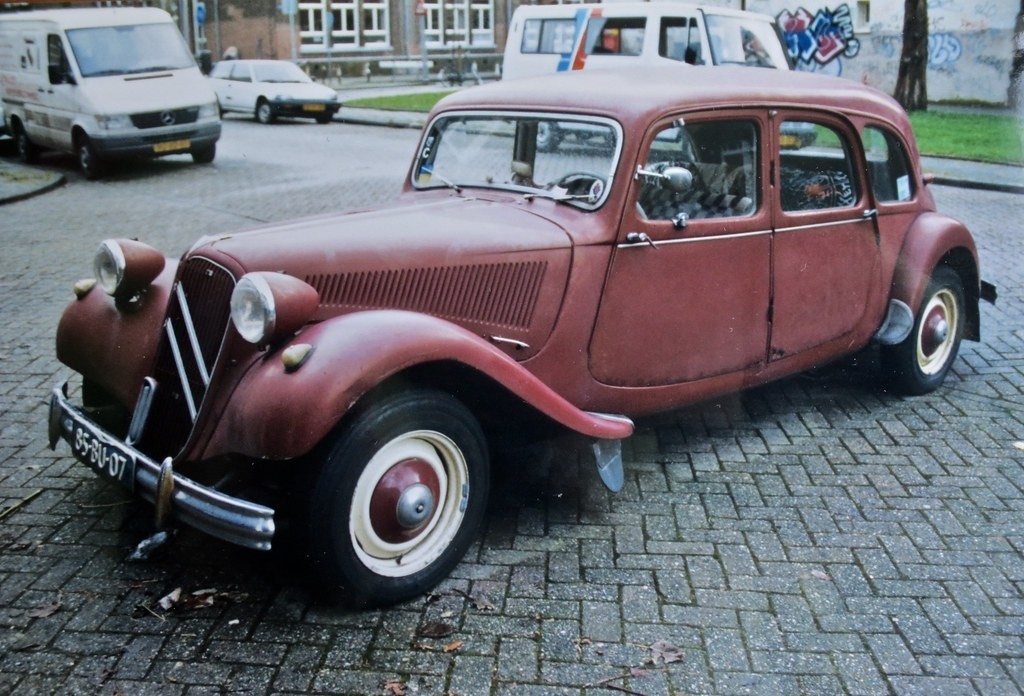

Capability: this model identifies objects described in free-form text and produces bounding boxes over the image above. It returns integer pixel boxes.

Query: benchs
[648,160,854,211]
[566,177,752,220]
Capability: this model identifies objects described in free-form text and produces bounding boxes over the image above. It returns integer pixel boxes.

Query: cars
[208,58,343,124]
[45,65,997,602]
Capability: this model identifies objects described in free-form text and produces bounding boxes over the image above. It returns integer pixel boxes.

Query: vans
[503,4,791,79]
[1,7,222,179]
